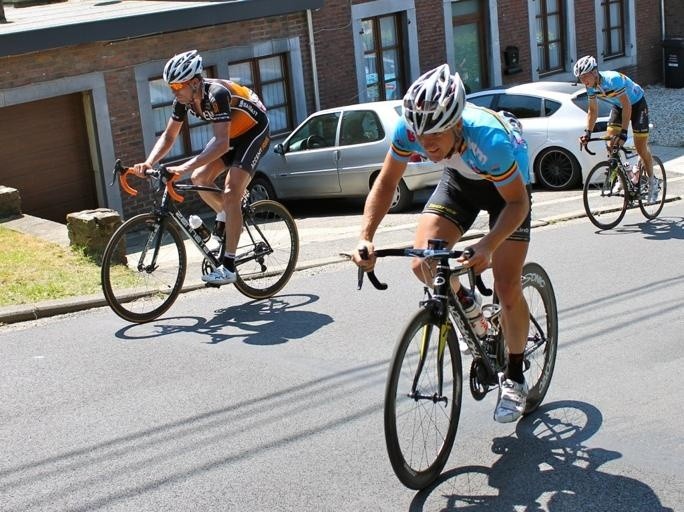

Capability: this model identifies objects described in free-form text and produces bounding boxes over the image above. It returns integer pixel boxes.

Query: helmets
[574,55,598,77]
[162,48,202,85]
[402,63,466,136]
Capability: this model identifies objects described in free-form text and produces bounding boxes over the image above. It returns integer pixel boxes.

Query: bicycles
[100,158,298,323]
[338,238,558,490]
[580,135,667,230]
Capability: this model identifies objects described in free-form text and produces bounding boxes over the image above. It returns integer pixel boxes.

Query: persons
[570,54,660,200]
[352,61,534,426]
[127,48,270,287]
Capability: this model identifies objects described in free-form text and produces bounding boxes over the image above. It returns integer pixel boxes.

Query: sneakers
[495,374,529,423]
[611,182,624,194]
[648,178,659,205]
[202,265,237,284]
[459,290,483,355]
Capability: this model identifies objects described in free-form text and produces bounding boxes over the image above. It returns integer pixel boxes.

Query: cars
[242,97,527,220]
[463,81,653,192]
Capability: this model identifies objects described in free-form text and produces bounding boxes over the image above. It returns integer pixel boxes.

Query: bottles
[622,162,634,180]
[632,163,639,183]
[214,212,227,240]
[462,293,490,341]
[187,214,212,241]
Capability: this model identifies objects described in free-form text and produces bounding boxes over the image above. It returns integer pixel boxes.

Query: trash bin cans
[664,37,684,88]
